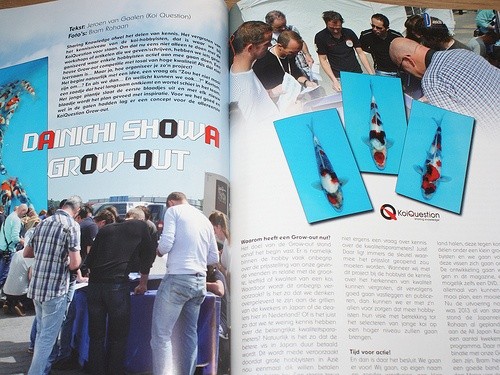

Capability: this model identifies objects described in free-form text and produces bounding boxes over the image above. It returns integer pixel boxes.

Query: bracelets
[304,80,307,88]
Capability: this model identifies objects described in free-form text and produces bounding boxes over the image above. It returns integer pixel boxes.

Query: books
[0,0,500,375]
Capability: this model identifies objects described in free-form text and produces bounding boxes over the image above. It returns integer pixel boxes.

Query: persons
[230,10,319,117]
[466,10,500,68]
[22,194,82,375]
[206,212,231,335]
[131,205,158,272]
[359,13,404,78]
[84,208,158,375]
[150,192,219,375]
[403,15,474,99]
[0,204,118,353]
[313,11,373,94]
[49,209,115,370]
[389,38,500,134]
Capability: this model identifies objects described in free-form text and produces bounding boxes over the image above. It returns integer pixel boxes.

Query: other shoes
[15,303,27,316]
[3,302,10,314]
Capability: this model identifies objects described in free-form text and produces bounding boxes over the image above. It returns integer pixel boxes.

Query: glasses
[397,54,410,73]
[371,24,385,30]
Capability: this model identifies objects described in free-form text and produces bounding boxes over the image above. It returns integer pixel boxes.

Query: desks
[62,281,224,375]
